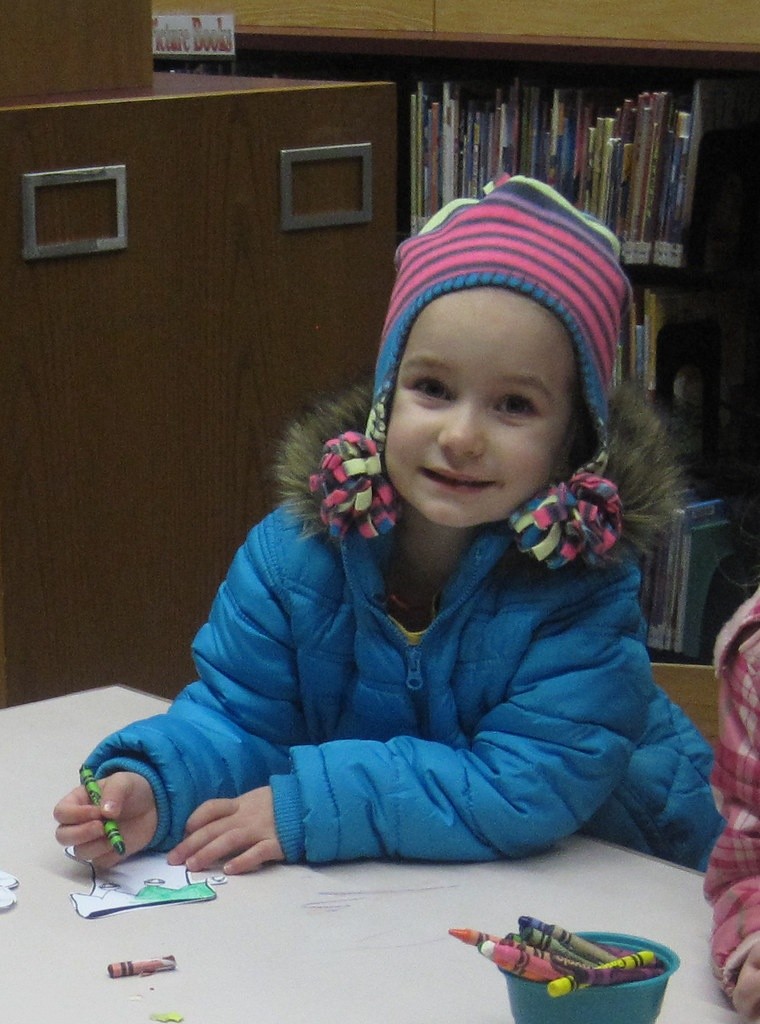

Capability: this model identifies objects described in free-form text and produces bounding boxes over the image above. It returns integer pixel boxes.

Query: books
[409,72,728,654]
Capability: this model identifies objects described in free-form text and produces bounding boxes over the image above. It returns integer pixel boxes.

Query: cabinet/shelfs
[390,41,760,743]
[1,77,390,703]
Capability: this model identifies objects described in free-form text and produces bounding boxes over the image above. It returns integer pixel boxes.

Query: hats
[306,172,624,570]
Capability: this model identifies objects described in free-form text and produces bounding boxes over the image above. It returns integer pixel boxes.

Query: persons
[702,580,760,1024]
[54,172,726,876]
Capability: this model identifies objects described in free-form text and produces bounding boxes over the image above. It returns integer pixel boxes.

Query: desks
[0,679,759,1024]
